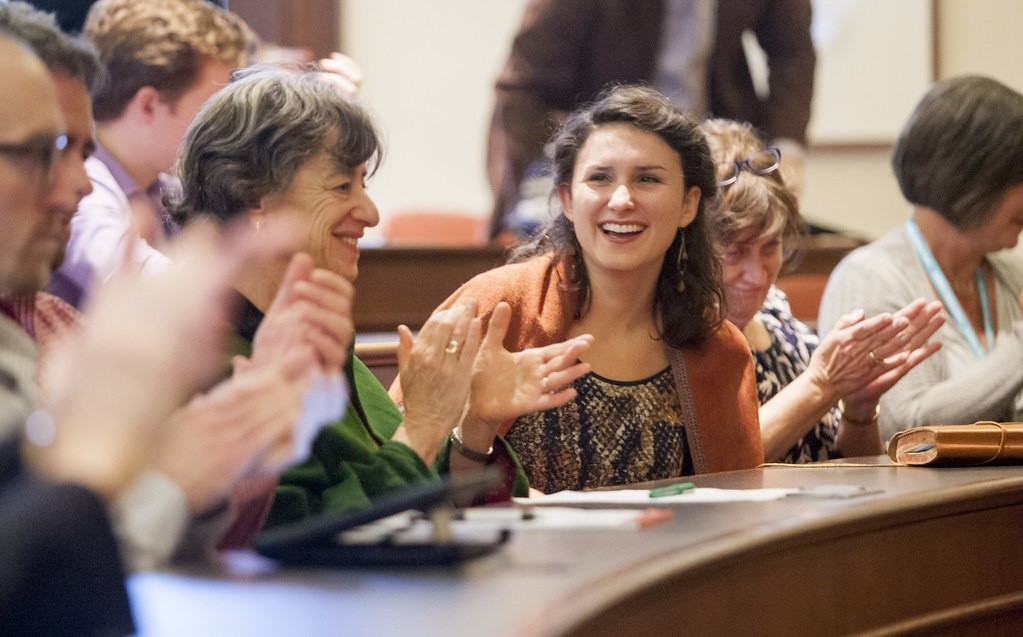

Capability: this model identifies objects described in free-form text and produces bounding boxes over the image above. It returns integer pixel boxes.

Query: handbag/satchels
[888,421,1023,466]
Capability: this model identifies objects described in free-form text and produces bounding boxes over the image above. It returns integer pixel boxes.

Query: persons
[815,73,1023,443]
[493,0,817,247]
[390,85,762,495]
[0,0,355,637]
[162,71,594,563]
[693,116,946,462]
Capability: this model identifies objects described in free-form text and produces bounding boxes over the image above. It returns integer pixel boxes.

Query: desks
[107,241,1023,637]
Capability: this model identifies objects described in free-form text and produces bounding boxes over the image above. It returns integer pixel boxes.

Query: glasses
[0,134,69,190]
[715,146,781,186]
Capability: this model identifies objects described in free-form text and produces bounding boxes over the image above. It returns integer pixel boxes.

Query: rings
[869,351,880,366]
[444,342,458,353]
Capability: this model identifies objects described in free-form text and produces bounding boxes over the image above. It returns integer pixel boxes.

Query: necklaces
[904,220,994,366]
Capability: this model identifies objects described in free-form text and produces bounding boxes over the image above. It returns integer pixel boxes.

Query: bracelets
[450,429,493,463]
[837,399,880,425]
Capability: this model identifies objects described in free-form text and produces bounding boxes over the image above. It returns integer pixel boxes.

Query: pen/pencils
[421,509,536,521]
[648,482,694,498]
